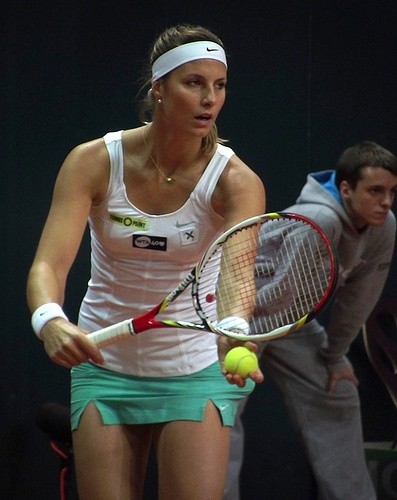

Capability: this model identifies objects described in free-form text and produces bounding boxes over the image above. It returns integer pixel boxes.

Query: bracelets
[215,317,250,344]
[31,303,68,340]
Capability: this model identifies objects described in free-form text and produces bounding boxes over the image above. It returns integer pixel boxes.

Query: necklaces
[143,122,203,182]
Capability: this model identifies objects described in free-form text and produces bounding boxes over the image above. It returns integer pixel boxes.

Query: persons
[223,141,397,500]
[27,25,265,500]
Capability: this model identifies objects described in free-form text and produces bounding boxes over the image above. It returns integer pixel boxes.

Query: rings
[351,376,352,380]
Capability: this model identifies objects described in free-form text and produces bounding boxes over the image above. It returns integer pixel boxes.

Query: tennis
[223,347,258,379]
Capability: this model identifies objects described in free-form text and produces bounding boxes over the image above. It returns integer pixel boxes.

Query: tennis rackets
[84,212,341,352]
